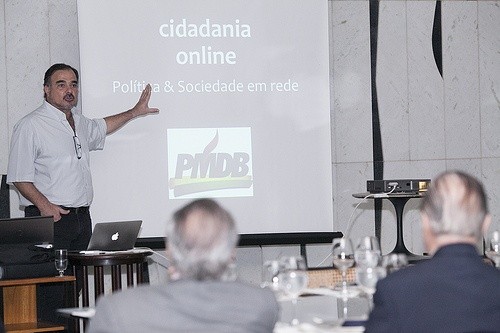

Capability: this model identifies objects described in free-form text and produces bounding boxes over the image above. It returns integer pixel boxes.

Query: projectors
[366,179,431,193]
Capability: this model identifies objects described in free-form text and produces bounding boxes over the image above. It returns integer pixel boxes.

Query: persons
[84,199,280,333]
[364,169,500,333]
[6,64,160,333]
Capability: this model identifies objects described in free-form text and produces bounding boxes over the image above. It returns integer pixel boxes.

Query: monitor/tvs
[0,215,53,265]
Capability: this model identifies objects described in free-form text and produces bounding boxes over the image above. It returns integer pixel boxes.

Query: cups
[489,230,500,252]
[354,235,380,269]
[260,260,281,298]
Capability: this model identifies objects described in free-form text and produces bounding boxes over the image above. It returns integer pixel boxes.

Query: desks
[59,250,155,333]
[0,274,77,333]
[353,193,428,259]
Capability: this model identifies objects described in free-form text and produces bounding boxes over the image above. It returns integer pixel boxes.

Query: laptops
[68,221,142,254]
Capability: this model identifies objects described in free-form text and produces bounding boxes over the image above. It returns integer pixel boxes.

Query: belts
[68,206,89,214]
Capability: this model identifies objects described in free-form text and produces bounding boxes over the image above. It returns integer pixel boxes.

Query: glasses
[73,135,82,160]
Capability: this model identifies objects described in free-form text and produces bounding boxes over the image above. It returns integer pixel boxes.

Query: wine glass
[280,256,312,329]
[332,238,358,296]
[355,250,386,311]
[54,249,68,277]
